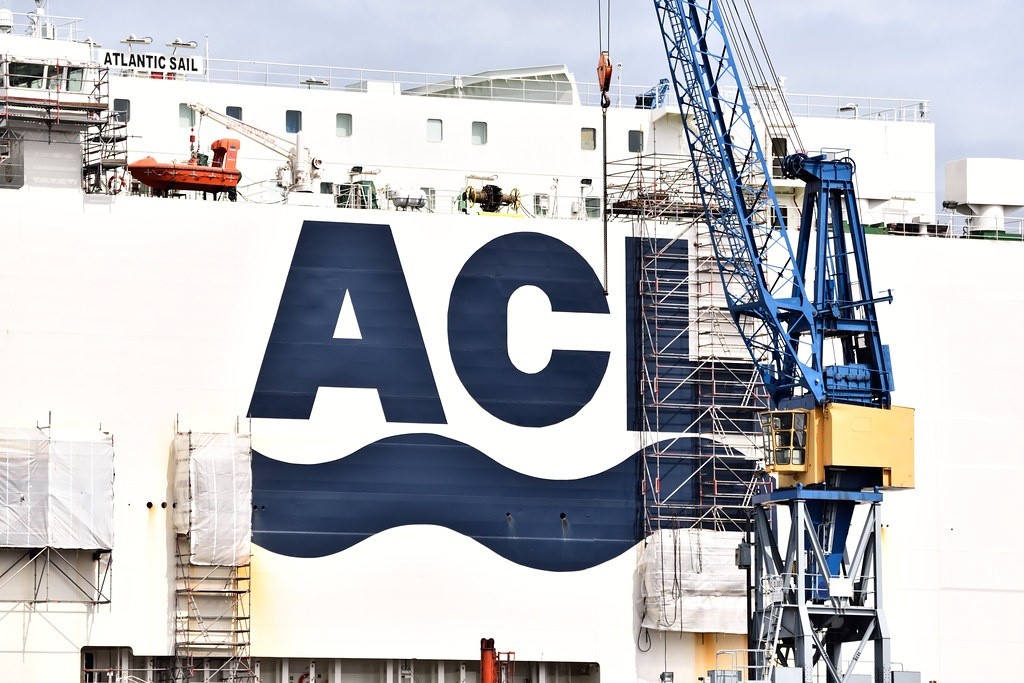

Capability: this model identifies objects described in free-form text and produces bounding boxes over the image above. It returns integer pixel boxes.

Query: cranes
[592,1,924,682]
[190,101,322,193]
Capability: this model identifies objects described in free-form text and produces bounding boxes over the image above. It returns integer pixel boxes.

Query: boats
[128,139,242,192]
[0,0,1024,683]
